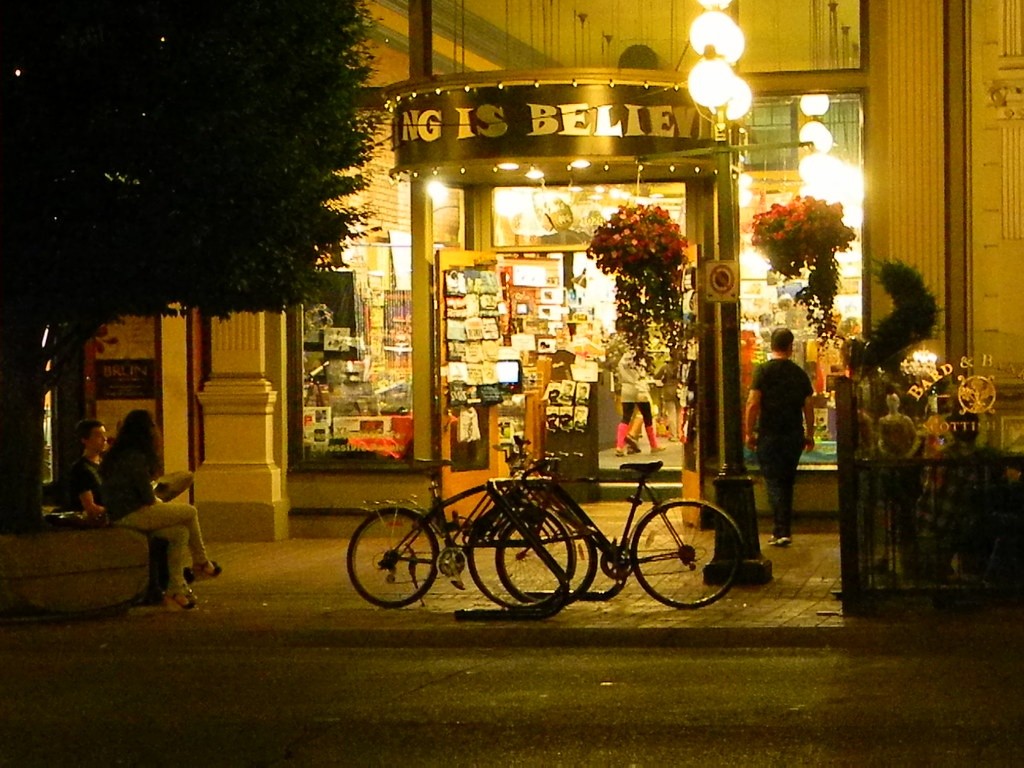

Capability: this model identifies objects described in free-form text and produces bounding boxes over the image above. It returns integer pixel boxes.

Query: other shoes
[651,447,666,455]
[615,447,624,456]
[768,531,791,545]
[184,568,196,583]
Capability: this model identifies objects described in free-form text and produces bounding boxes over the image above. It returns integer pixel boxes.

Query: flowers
[749,195,856,351]
[584,208,694,365]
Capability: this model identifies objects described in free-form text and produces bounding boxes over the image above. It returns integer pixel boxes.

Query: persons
[934,406,1015,575]
[606,331,692,457]
[741,298,859,397]
[74,410,221,610]
[879,392,921,565]
[746,328,815,545]
[831,340,886,451]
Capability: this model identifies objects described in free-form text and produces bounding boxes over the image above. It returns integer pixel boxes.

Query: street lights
[687,0,774,585]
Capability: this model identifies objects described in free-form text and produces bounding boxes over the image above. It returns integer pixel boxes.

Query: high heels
[162,591,199,612]
[192,561,222,581]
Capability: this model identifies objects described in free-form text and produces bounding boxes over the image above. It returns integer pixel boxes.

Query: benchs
[0,527,150,619]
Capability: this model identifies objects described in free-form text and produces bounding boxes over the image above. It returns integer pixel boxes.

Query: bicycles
[346,435,749,612]
